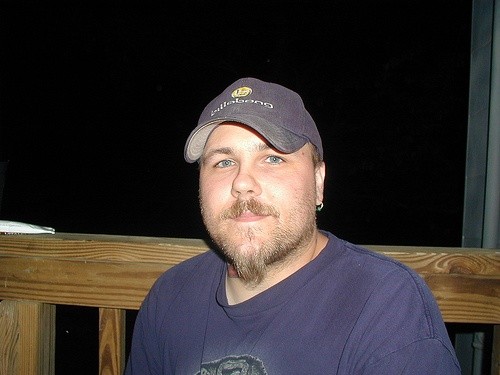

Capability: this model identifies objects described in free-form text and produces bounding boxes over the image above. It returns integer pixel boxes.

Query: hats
[185,78,323,164]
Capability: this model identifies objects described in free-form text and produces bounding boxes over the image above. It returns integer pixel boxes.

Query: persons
[116,78,462,375]
